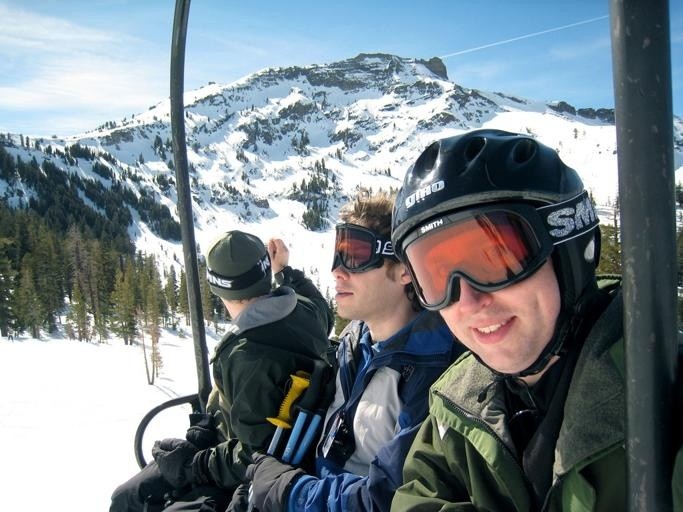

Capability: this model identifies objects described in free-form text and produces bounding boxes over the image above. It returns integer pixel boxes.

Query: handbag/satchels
[138,475,191,504]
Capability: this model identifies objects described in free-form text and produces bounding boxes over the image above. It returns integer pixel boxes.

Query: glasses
[400,191,600,312]
[331,224,399,273]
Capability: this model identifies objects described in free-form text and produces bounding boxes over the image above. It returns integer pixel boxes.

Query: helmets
[391,129,600,306]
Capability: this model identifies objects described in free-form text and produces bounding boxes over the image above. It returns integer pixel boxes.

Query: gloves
[225,483,247,512]
[187,413,216,449]
[246,452,307,512]
[152,439,214,491]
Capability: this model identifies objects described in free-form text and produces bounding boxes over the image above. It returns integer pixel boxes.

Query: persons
[390,130,683,512]
[227,196,466,512]
[110,231,335,512]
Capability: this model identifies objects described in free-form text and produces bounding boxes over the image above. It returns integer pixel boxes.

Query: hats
[205,230,271,299]
